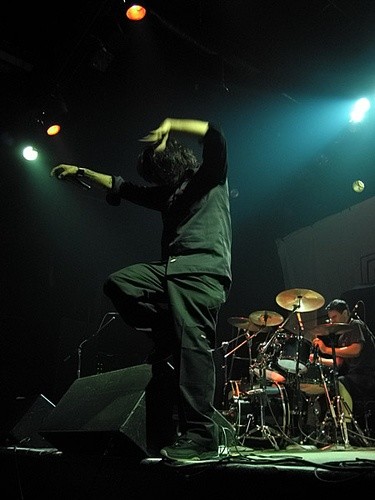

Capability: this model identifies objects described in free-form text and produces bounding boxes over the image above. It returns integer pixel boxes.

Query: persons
[253,328,317,444]
[49,119,233,461]
[308,299,375,447]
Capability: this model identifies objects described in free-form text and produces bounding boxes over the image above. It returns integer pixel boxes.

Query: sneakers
[148,330,171,355]
[160,436,218,461]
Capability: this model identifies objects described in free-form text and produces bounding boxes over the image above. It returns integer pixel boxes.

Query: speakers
[0,362,236,463]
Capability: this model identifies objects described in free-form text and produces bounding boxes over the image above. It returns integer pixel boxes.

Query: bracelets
[76,167,85,176]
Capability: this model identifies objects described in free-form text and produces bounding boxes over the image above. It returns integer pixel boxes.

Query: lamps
[28,106,63,139]
[117,0,149,25]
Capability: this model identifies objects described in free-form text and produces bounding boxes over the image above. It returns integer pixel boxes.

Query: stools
[352,396,375,432]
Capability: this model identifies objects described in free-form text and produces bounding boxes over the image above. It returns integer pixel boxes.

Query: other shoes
[329,423,343,443]
[316,425,331,444]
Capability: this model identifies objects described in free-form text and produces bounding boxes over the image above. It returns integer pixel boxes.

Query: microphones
[296,312,304,331]
[347,303,359,323]
[107,312,120,317]
[55,168,92,191]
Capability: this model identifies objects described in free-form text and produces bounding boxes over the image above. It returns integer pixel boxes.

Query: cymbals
[249,310,284,326]
[310,323,356,337]
[227,317,273,333]
[276,288,325,313]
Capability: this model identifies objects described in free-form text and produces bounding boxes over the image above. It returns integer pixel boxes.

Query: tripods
[233,299,369,452]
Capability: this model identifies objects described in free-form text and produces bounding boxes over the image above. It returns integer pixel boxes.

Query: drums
[252,354,289,383]
[274,334,315,373]
[254,340,288,381]
[245,374,281,396]
[223,381,291,450]
[296,361,332,394]
